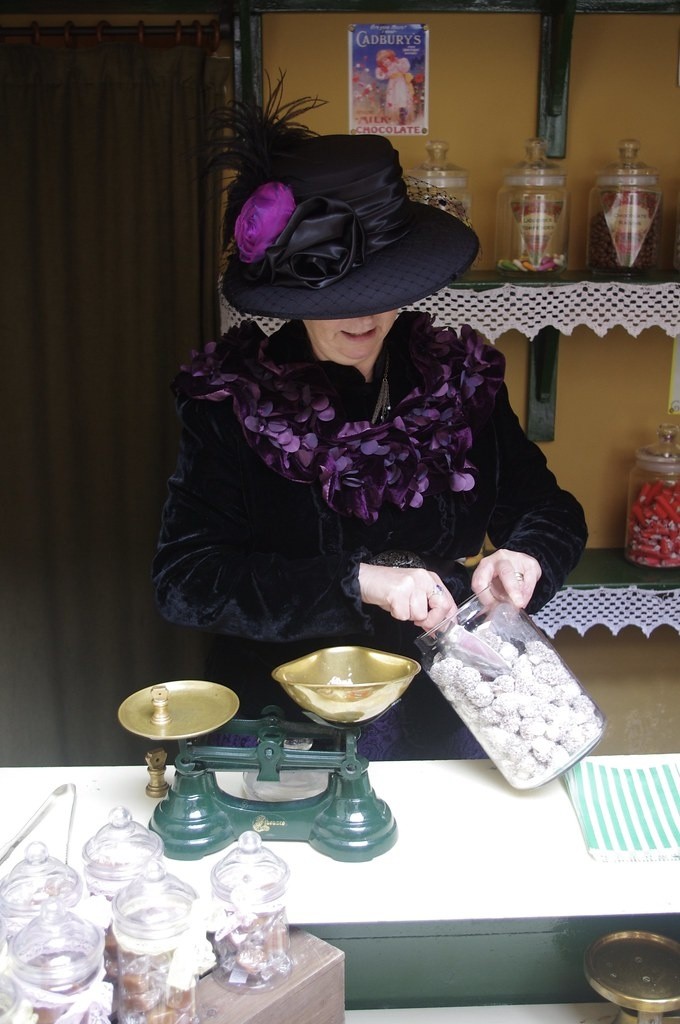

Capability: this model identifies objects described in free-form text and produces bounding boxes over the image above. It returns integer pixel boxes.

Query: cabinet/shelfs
[217,263,679,636]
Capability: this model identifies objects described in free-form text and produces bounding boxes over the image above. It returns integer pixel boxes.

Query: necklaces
[370,347,391,426]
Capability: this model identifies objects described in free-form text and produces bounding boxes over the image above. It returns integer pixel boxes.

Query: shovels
[436,618,513,678]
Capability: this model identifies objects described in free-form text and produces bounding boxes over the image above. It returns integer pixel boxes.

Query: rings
[428,584,444,599]
[515,572,524,581]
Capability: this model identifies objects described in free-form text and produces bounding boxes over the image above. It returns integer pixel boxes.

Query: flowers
[216,180,371,289]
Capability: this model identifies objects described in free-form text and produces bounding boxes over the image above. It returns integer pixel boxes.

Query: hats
[189,67,482,338]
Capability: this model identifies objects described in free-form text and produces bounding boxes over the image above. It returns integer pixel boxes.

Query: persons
[152,134,588,761]
